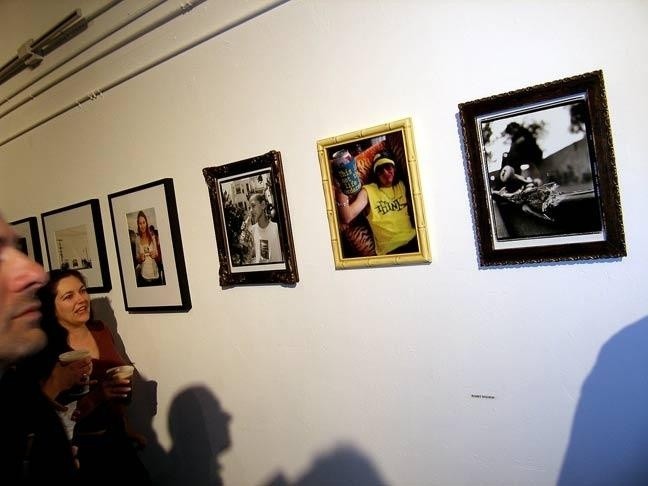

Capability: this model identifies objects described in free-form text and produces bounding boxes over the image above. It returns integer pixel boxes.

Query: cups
[107,364,135,404]
[58,349,93,398]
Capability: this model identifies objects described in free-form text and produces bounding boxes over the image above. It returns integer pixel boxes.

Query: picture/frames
[455,65,628,273]
[4,215,44,274]
[104,173,193,314]
[38,196,114,296]
[311,112,434,273]
[202,142,302,291]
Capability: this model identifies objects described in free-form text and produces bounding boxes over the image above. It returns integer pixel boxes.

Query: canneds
[332,149,363,195]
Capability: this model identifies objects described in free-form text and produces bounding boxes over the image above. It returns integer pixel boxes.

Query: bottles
[238,213,253,247]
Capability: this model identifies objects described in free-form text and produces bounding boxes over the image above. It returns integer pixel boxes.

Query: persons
[247,194,283,263]
[31,267,152,486]
[128,211,164,286]
[0,215,83,486]
[494,123,542,194]
[333,151,419,255]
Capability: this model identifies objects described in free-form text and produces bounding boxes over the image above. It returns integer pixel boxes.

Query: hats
[374,153,396,171]
